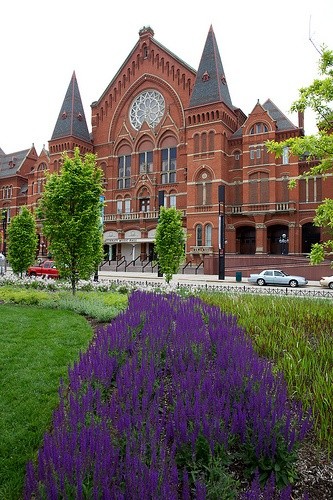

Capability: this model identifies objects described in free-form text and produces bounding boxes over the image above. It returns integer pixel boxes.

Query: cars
[26,261,60,281]
[319,276,333,289]
[248,270,308,288]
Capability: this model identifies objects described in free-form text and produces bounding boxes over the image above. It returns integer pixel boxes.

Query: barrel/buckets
[236,272,242,281]
